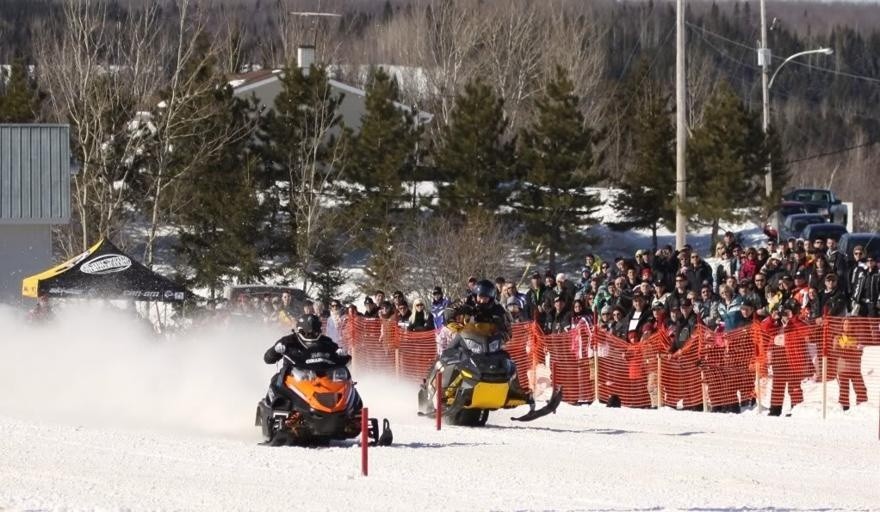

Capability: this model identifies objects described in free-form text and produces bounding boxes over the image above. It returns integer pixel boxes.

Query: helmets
[473,280,496,297]
[295,314,323,342]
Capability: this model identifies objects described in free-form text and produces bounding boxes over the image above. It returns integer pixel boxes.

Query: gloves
[275,342,286,352]
[336,346,346,355]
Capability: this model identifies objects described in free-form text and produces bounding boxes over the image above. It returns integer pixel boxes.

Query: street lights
[755,48,836,199]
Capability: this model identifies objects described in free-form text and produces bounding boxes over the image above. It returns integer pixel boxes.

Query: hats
[740,300,752,311]
[633,269,691,310]
[532,269,568,302]
[779,236,866,282]
[433,286,443,295]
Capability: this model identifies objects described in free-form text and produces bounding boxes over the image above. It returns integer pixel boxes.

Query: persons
[27,291,54,320]
[830,199,848,228]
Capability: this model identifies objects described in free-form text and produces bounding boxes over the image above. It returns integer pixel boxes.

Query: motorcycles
[254,339,394,448]
[416,306,563,428]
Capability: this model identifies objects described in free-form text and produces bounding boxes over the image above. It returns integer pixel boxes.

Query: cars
[764,189,878,276]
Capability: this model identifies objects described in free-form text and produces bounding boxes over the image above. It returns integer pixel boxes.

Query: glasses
[394,294,423,310]
